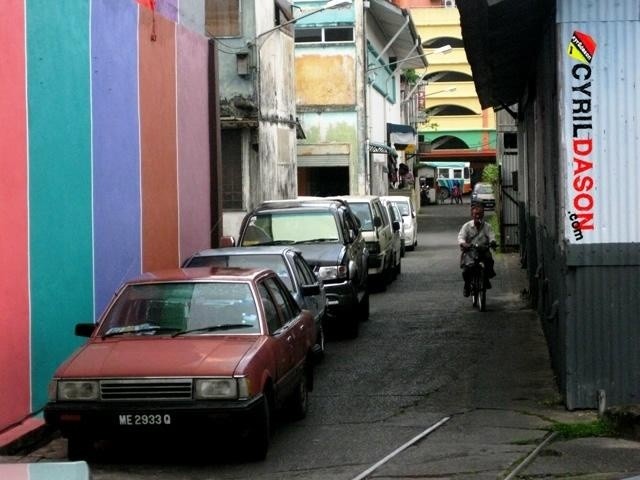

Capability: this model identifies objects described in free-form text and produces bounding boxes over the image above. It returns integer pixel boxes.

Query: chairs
[159,302,190,331]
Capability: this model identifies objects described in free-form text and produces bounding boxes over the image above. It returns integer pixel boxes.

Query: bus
[414,162,473,199]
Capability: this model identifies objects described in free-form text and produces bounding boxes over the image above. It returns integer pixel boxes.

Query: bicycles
[461,243,499,311]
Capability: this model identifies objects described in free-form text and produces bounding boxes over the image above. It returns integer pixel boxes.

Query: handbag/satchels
[460,251,466,268]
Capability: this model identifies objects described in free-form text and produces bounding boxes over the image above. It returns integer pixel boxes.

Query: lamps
[235,50,251,77]
[255,1,351,49]
[366,43,453,71]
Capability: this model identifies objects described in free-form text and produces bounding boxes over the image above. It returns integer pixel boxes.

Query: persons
[457,204,496,297]
[420,181,462,205]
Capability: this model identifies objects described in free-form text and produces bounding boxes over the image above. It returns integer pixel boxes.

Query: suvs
[219,196,417,338]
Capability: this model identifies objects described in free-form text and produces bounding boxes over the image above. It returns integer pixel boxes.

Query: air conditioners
[444,1,456,9]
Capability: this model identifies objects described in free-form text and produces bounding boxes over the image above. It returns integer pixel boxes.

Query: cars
[472,182,495,208]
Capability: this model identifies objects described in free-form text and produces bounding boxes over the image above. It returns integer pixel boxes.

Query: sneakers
[487,279,492,289]
[463,284,470,297]
